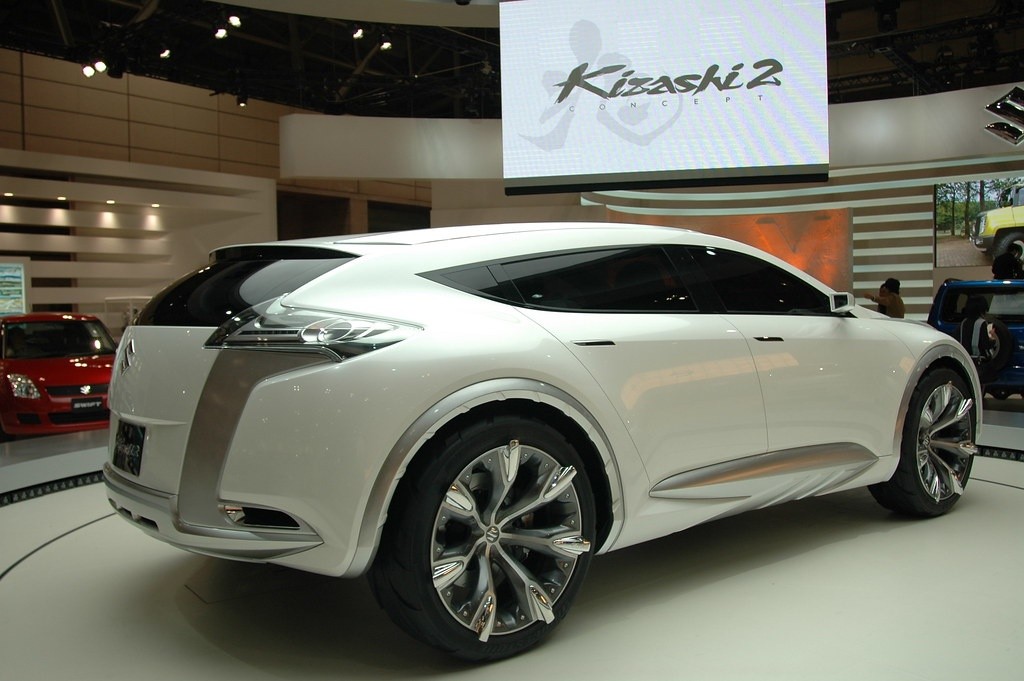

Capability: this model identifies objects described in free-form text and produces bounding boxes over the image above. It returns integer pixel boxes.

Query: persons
[991,243,1020,279]
[956,296,995,399]
[863,278,906,319]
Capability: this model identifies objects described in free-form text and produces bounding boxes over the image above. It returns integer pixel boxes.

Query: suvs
[924,274,1024,401]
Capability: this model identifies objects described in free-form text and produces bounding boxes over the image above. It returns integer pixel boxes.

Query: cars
[971,185,1023,260]
[102,224,985,667]
[0,313,116,437]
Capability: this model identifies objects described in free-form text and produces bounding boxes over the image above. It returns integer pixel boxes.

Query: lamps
[236,93,247,108]
[107,54,127,79]
[348,22,365,40]
[377,32,392,51]
